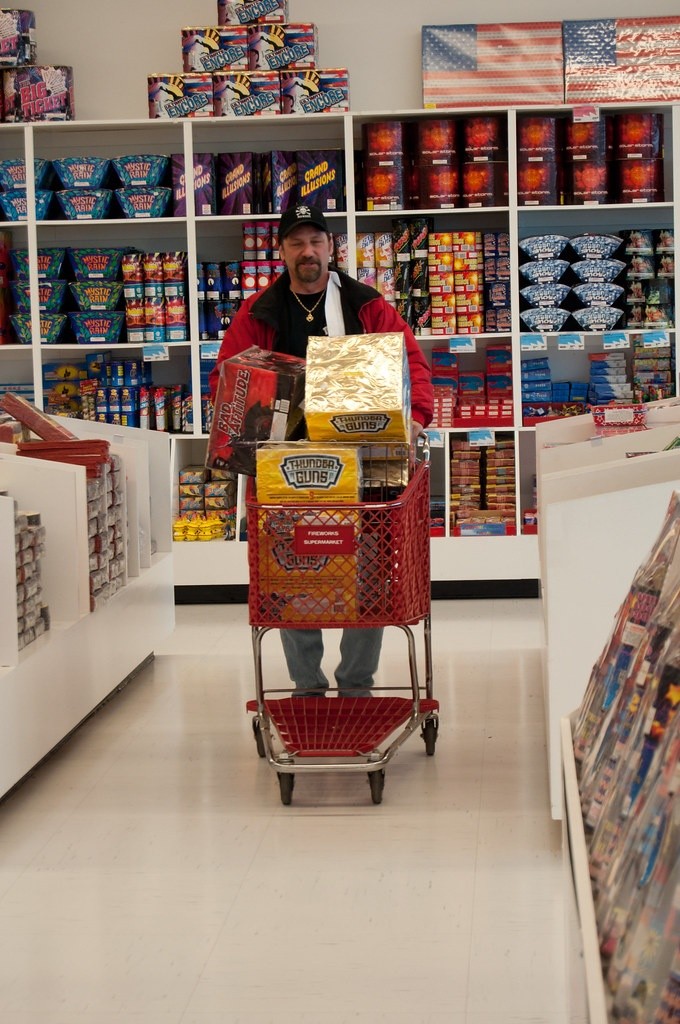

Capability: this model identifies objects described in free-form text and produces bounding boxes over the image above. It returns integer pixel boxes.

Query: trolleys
[242,429,444,805]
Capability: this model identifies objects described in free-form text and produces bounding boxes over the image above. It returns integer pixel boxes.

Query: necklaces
[290,285,327,322]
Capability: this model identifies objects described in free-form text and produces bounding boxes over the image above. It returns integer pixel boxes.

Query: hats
[277,205,328,245]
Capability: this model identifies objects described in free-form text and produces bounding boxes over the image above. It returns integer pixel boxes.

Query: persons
[209,205,435,697]
